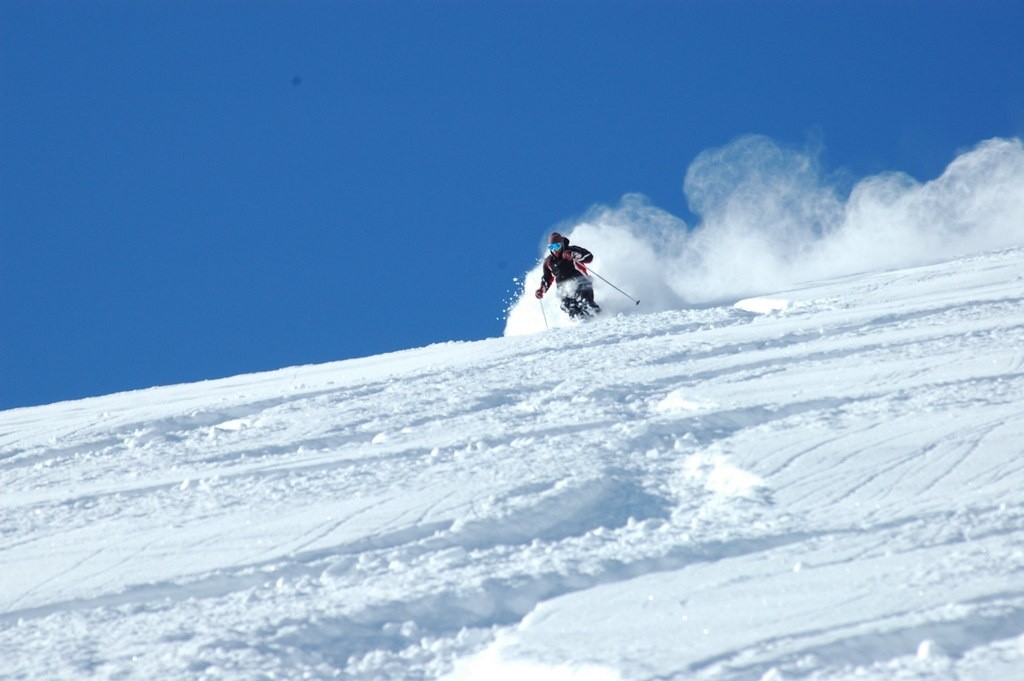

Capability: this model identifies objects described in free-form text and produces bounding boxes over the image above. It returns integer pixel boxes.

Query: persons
[535,231,602,321]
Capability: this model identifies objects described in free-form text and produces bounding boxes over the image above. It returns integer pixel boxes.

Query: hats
[548,232,561,244]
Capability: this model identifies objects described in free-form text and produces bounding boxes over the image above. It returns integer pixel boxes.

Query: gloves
[535,289,543,299]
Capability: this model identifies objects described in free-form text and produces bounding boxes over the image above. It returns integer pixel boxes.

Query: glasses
[546,242,562,251]
